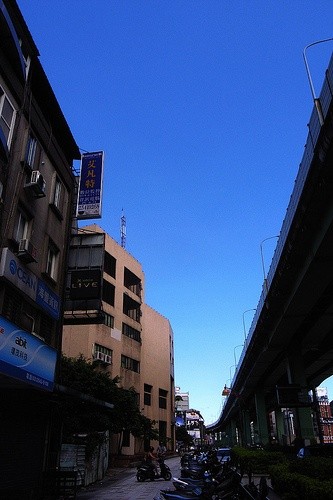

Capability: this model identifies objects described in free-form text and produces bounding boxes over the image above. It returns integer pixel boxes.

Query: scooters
[136,456,172,482]
[153,450,244,500]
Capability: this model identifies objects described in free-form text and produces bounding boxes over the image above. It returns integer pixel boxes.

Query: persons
[145,440,166,480]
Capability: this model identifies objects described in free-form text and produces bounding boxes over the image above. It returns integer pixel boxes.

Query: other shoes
[151,479,155,482]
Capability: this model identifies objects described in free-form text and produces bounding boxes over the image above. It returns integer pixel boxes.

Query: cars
[217,448,232,462]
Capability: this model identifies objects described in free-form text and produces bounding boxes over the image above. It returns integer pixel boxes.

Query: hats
[160,442,163,445]
[149,446,154,450]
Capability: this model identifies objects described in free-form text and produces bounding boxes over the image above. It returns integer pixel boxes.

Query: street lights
[259,235,280,279]
[233,344,244,369]
[243,308,256,339]
[230,364,238,383]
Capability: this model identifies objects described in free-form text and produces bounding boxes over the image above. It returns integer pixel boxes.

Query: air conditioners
[30,169,47,193]
[18,238,36,259]
